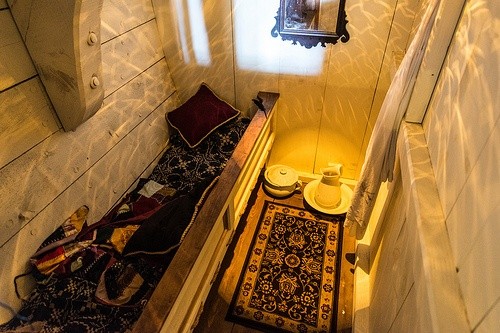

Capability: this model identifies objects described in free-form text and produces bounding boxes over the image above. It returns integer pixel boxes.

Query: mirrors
[284,0,340,32]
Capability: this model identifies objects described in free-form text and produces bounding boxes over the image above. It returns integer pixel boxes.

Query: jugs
[314,163,343,208]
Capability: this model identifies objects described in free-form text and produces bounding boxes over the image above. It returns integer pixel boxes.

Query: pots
[263,164,303,198]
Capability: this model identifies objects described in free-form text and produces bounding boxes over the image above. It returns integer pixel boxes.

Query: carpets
[225,199,346,333]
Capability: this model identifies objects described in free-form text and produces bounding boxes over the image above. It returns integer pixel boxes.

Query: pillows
[163,80,242,149]
[120,175,219,257]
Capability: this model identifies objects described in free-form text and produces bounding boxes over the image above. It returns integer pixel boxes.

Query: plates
[304,179,354,215]
